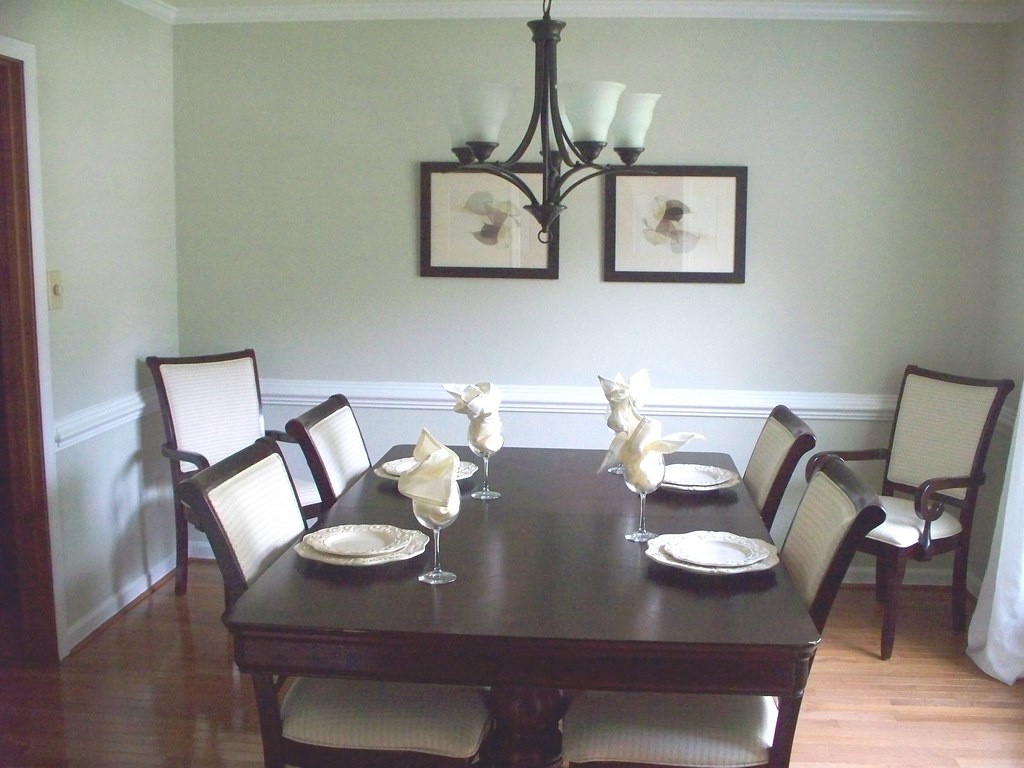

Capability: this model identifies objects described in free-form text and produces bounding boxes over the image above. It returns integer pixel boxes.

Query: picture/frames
[420,161,561,280]
[604,165,748,283]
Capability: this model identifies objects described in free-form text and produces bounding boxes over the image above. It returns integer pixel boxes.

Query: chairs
[803,364,1015,659]
[178,400,886,768]
[146,349,323,611]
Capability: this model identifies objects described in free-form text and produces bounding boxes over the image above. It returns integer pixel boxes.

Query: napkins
[440,380,503,454]
[597,417,707,488]
[398,428,459,523]
[598,375,644,435]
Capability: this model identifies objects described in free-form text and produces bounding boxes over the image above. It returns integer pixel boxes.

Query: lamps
[432,0,664,244]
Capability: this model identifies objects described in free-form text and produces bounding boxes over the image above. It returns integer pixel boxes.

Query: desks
[222,445,820,768]
